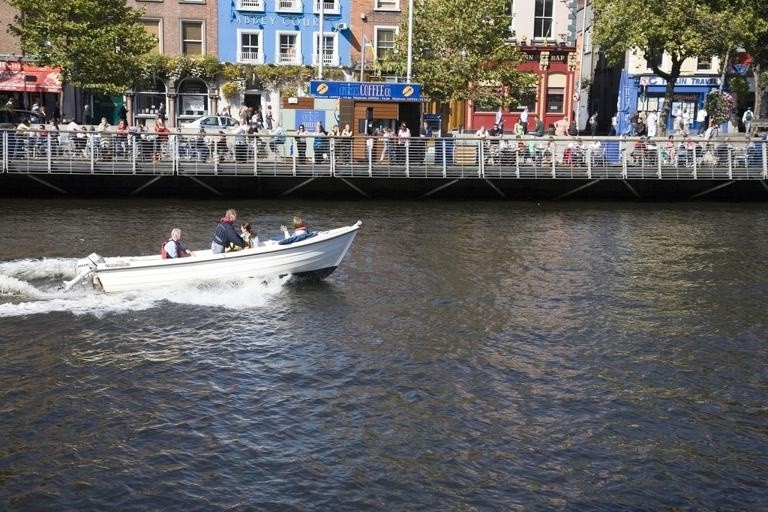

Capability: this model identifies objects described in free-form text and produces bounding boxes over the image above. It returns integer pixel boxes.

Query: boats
[60,220,363,296]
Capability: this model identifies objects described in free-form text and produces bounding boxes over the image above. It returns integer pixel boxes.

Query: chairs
[1,123,768,170]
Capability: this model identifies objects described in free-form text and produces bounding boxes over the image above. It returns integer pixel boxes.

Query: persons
[162,228,191,259]
[211,209,248,254]
[476,106,602,166]
[3,99,63,158]
[367,121,432,167]
[279,217,307,239]
[240,222,258,248]
[70,100,170,163]
[294,121,353,165]
[608,108,718,167]
[741,107,756,147]
[174,104,286,164]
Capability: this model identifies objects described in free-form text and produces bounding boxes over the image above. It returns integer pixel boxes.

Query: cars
[0,108,88,158]
[179,115,268,142]
